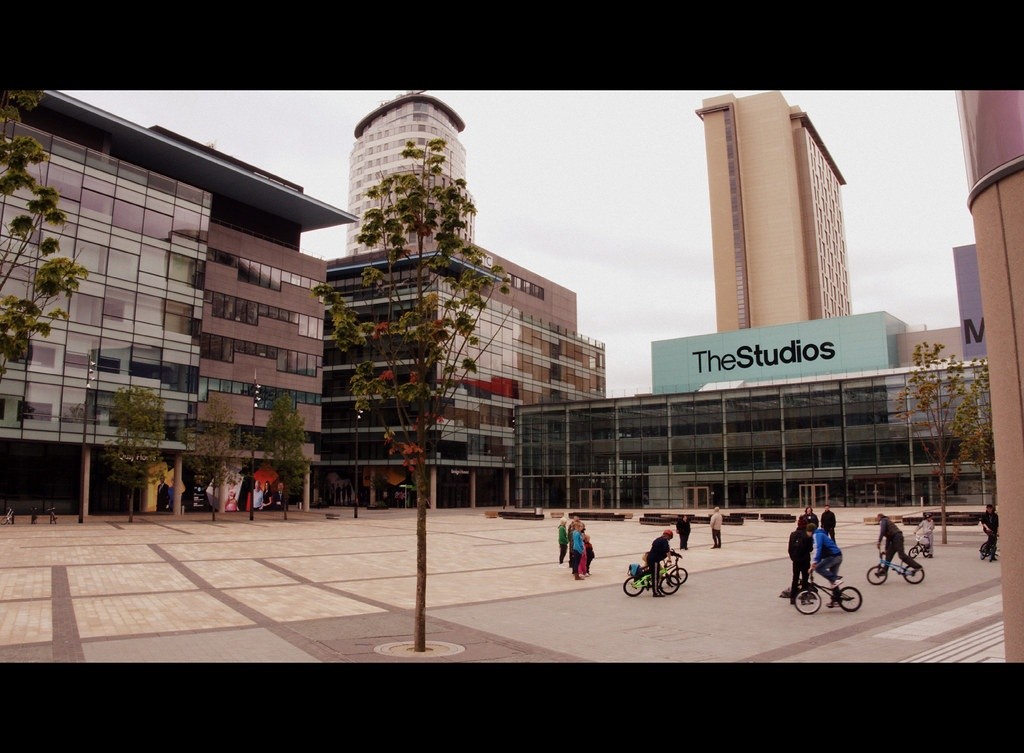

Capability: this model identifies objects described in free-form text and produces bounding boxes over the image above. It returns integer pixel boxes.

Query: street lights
[250,366,262,521]
[78,354,97,527]
[353,407,363,520]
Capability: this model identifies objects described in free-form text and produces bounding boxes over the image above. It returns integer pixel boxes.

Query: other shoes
[559,562,567,568]
[875,569,886,575]
[575,572,592,579]
[659,592,665,597]
[928,553,932,557]
[986,550,991,554]
[911,565,922,572]
[993,557,997,560]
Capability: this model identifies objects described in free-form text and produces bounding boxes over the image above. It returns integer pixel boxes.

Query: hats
[663,529,673,539]
[925,513,932,517]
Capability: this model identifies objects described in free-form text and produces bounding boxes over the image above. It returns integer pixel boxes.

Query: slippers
[826,602,839,606]
[832,576,847,588]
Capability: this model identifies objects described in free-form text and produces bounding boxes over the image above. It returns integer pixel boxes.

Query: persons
[156,474,174,512]
[874,514,923,576]
[225,480,240,512]
[710,507,723,549]
[805,523,843,607]
[330,481,352,505]
[643,551,664,584]
[820,505,836,544]
[644,530,673,597]
[789,517,813,604]
[676,515,690,549]
[558,516,594,581]
[253,480,284,511]
[802,506,819,548]
[915,512,934,558]
[981,504,999,560]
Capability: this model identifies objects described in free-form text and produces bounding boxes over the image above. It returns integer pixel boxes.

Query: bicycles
[867,545,925,585]
[980,530,999,562]
[48,505,58,524]
[908,532,929,559]
[794,569,863,615]
[29,504,38,524]
[622,560,680,597]
[0,503,16,525]
[981,541,991,557]
[662,549,688,586]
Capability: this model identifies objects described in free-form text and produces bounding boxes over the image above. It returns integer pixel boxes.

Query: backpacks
[791,530,806,556]
